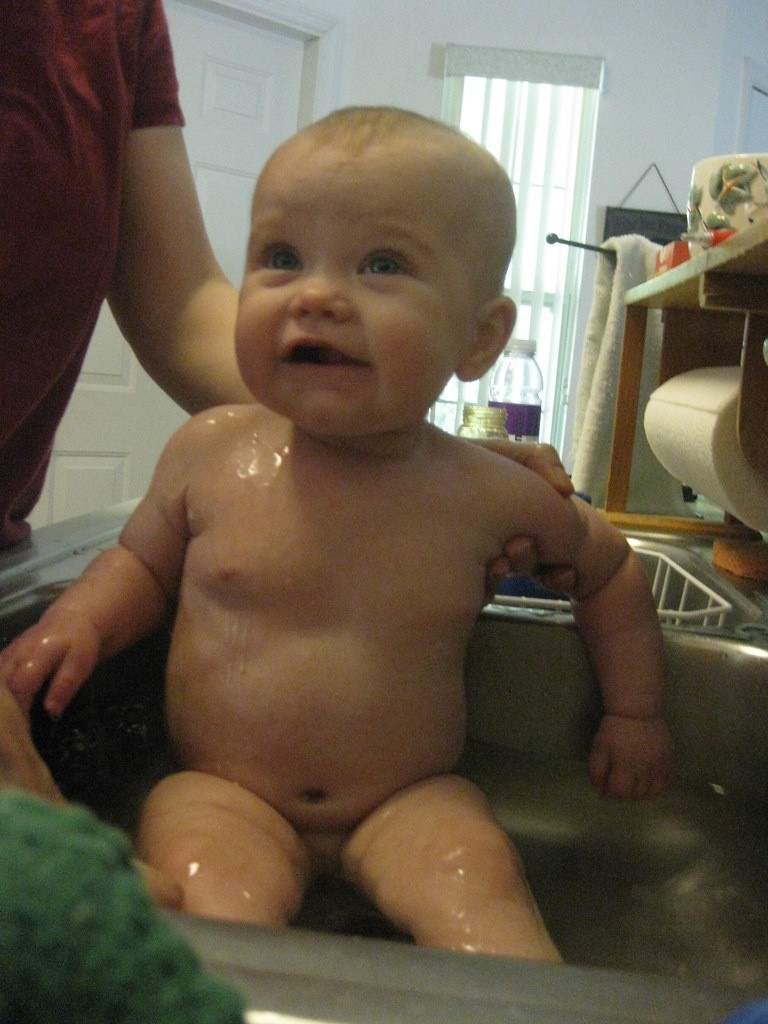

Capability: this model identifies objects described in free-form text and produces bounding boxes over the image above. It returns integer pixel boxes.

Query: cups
[686,152,768,258]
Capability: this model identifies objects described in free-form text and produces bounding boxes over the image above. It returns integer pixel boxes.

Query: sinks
[487,529,764,629]
[0,600,768,1024]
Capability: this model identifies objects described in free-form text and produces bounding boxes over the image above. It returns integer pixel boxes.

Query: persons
[1,105,667,965]
[0,0,578,909]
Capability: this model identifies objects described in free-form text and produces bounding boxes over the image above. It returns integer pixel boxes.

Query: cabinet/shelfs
[594,208,767,539]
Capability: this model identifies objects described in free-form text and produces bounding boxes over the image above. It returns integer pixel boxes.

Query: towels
[571,232,678,514]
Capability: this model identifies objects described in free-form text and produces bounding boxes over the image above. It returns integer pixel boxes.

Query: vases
[687,155,768,258]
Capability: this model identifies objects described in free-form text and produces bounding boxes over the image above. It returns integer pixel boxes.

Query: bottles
[455,402,509,440]
[488,337,544,443]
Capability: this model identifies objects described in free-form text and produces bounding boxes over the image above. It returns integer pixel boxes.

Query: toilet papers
[642,362,767,529]
